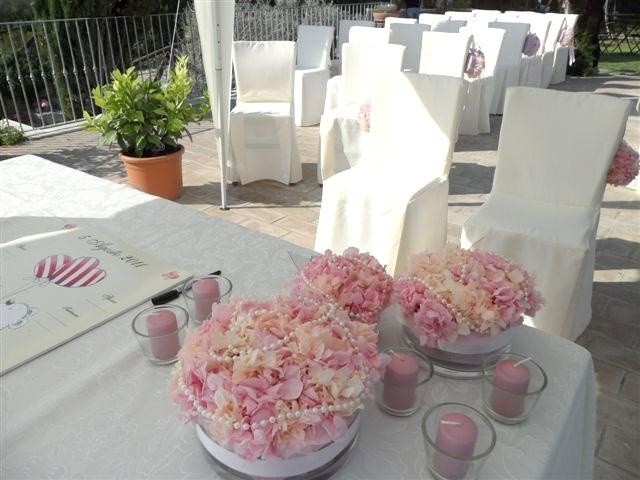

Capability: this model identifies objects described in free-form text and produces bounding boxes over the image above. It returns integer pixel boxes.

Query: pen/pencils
[150,270,222,307]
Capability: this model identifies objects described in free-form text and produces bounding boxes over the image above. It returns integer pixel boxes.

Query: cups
[375,347,433,416]
[484,353,546,424]
[185,274,231,326]
[420,403,497,480]
[131,303,193,365]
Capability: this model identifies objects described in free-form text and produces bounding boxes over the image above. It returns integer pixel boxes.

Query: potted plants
[82,53,213,202]
[370,2,404,22]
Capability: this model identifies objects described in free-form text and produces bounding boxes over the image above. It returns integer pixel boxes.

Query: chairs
[520,15,550,89]
[461,86,632,342]
[349,26,388,43]
[384,17,417,29]
[446,11,475,21]
[487,22,528,124]
[474,9,502,21]
[316,71,466,284]
[231,41,299,187]
[457,26,505,136]
[390,23,429,74]
[551,14,577,84]
[543,12,566,87]
[419,13,451,31]
[340,42,406,73]
[417,32,471,148]
[295,25,331,123]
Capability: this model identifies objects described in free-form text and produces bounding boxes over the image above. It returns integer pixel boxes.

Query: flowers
[170,292,376,458]
[465,48,485,78]
[292,238,391,325]
[396,242,544,349]
[524,33,542,55]
[559,24,577,66]
[607,135,640,189]
